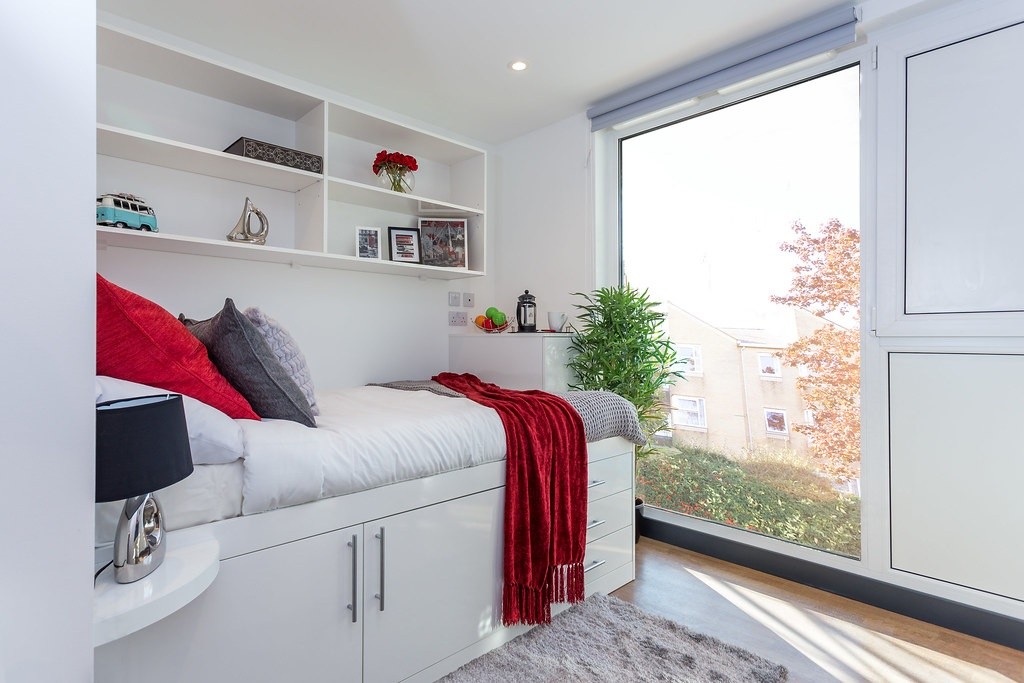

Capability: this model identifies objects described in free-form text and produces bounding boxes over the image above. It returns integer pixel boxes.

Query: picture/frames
[387,227,422,264]
[417,217,468,269]
[355,226,382,260]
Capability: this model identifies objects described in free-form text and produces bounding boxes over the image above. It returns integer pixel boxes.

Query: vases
[376,163,415,195]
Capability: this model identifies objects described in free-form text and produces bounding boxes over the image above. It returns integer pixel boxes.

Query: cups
[548,311,568,332]
[517,289,536,332]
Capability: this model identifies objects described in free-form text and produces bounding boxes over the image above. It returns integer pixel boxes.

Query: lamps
[96,394,194,584]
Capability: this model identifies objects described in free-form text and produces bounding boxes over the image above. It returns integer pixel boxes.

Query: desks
[95,540,219,646]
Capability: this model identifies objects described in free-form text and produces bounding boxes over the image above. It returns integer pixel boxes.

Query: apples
[475,307,507,329]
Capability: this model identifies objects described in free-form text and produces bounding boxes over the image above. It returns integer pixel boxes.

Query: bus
[96,192,159,233]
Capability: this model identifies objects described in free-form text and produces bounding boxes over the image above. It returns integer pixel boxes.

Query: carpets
[432,591,789,683]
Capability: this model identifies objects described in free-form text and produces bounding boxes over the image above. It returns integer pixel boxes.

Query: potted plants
[567,273,689,543]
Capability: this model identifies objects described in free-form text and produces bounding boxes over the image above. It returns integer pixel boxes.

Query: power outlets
[448,311,468,326]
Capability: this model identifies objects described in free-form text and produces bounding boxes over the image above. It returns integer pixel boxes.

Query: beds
[96,372,646,562]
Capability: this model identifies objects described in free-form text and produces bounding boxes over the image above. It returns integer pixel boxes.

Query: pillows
[96,273,264,422]
[95,375,246,464]
[242,306,321,419]
[178,297,318,430]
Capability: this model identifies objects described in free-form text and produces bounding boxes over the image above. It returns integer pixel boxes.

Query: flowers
[373,150,419,193]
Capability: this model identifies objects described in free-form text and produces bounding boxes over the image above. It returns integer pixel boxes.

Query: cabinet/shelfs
[94,485,507,683]
[96,19,488,280]
[555,451,636,610]
[448,332,585,395]
